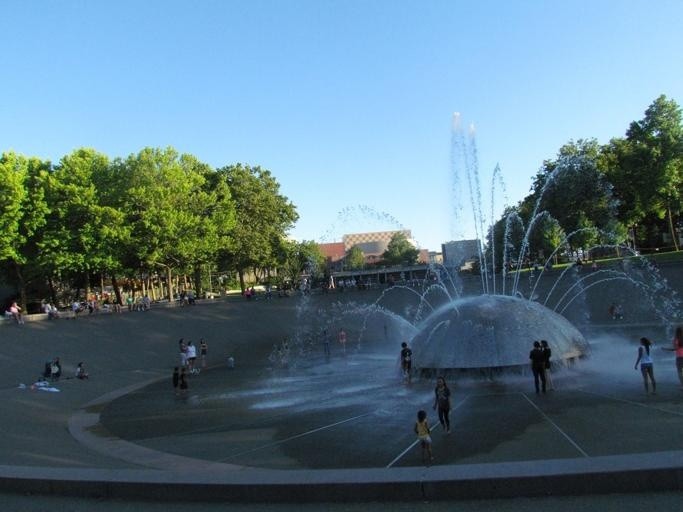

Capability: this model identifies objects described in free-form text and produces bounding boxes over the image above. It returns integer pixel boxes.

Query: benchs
[0,299,207,326]
[239,279,375,302]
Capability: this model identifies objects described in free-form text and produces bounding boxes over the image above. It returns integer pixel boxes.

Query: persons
[41,289,196,320]
[529,340,554,394]
[10,302,23,325]
[633,338,657,396]
[499,255,600,274]
[660,325,682,385]
[43,356,88,382]
[242,270,439,302]
[432,376,452,435]
[608,302,624,320]
[413,409,435,462]
[384,324,412,375]
[173,335,236,395]
[273,327,347,356]
